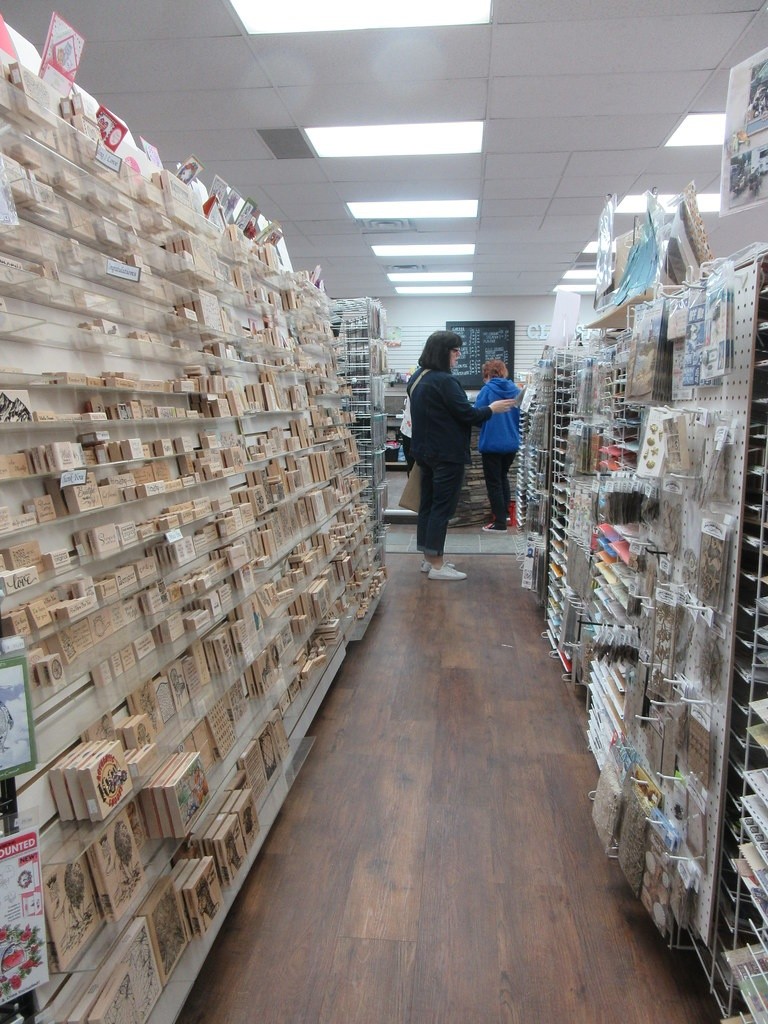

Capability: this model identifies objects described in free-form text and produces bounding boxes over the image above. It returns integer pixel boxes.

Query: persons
[405,330,515,583]
[399,396,416,477]
[474,360,523,533]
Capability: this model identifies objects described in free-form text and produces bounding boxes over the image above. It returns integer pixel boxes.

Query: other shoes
[482,520,508,533]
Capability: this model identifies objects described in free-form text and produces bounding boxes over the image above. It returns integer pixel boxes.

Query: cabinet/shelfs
[0,50,768,1024]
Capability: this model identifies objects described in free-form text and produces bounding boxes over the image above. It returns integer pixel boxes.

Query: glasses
[451,347,461,355]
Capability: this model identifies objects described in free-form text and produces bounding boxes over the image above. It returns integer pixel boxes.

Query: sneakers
[421,559,456,572]
[427,561,467,580]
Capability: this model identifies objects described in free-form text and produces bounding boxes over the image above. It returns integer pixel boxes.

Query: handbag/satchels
[398,460,422,512]
